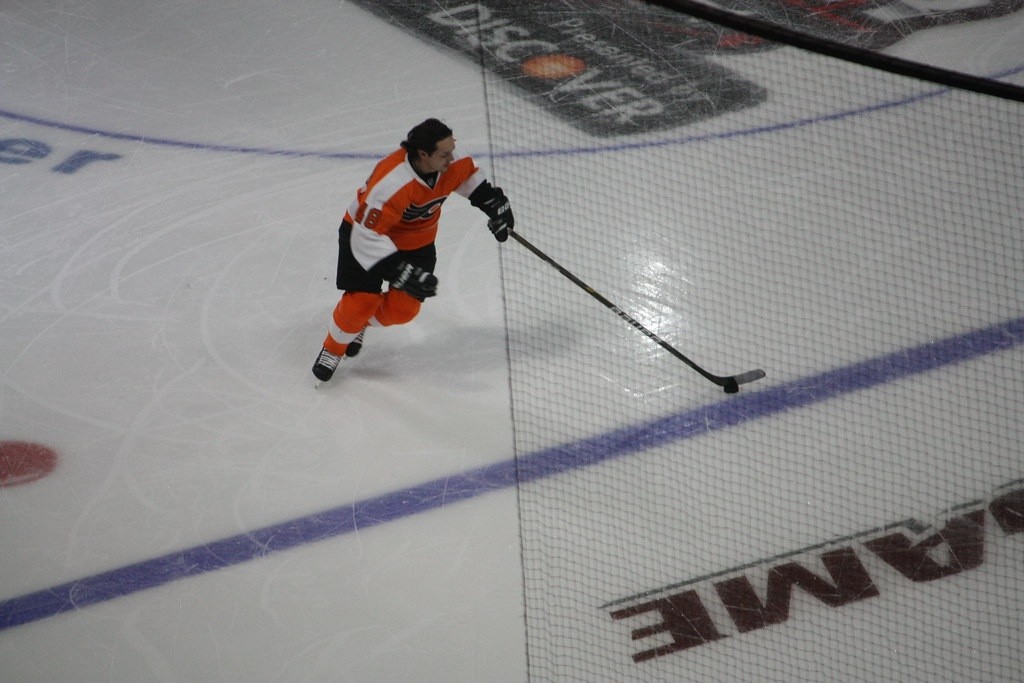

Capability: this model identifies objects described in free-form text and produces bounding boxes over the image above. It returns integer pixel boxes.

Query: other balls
[724,387,740,393]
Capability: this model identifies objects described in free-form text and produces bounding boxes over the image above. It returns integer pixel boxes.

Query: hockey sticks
[507,227,766,386]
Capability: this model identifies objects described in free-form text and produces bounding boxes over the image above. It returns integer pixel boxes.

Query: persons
[312,118,515,390]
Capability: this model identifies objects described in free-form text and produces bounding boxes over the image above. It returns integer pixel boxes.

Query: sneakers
[312,347,341,388]
[343,327,367,360]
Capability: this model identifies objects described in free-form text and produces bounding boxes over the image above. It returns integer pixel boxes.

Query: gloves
[390,260,437,297]
[471,187,515,241]
